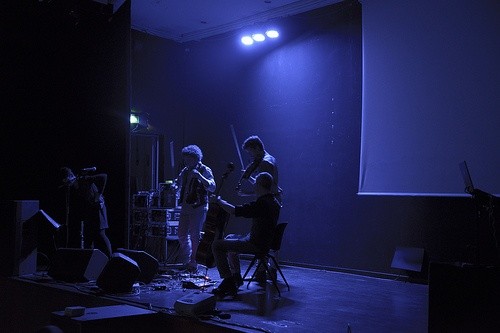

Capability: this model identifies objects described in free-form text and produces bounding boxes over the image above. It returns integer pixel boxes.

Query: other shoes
[190,260,197,270]
[177,262,193,272]
[254,269,267,280]
[211,280,237,295]
[266,270,277,280]
[232,275,243,286]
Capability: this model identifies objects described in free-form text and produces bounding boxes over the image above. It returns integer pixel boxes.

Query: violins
[240,154,260,183]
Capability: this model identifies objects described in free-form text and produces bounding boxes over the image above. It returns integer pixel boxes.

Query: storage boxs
[132,191,181,237]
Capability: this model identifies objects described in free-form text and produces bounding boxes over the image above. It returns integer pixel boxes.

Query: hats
[250,172,273,185]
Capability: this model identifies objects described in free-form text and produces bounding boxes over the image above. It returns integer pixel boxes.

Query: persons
[170,144,217,267]
[239,135,281,280]
[210,171,281,297]
[57,167,113,257]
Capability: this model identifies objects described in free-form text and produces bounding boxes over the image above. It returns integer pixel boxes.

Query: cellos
[196,162,235,293]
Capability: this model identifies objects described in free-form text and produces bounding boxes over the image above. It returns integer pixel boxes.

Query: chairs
[239,221,291,297]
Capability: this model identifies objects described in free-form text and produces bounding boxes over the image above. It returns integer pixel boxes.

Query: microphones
[227,161,235,172]
[83,167,97,171]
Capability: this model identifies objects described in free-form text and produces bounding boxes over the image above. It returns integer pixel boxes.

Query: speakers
[427,257,500,333]
[7,200,183,294]
